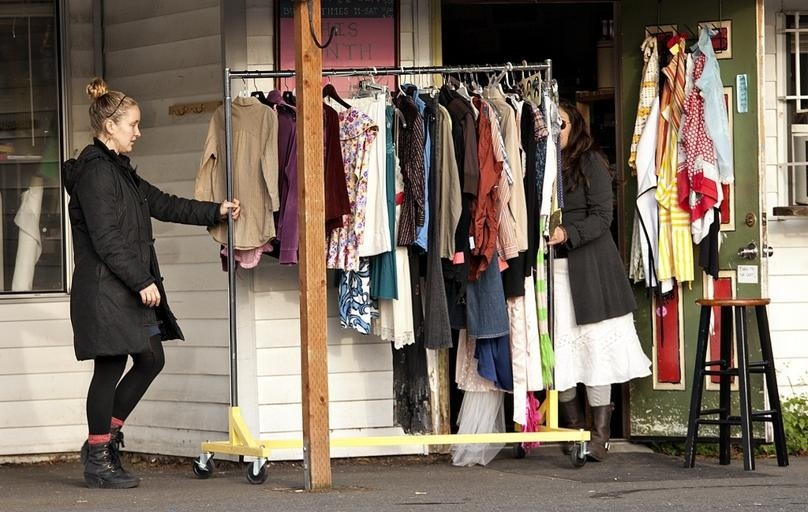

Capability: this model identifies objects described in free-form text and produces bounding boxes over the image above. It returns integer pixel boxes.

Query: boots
[559,397,582,454]
[585,402,615,462]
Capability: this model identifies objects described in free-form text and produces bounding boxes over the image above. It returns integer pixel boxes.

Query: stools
[680,298,792,468]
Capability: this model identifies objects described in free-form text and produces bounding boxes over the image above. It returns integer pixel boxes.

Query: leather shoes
[81,442,139,489]
[79,427,125,472]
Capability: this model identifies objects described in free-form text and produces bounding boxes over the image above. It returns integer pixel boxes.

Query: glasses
[561,120,571,129]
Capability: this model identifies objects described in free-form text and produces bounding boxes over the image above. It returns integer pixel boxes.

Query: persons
[547,101,653,461]
[61,79,240,489]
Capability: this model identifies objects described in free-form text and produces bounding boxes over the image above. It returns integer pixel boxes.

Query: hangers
[235,58,539,128]
